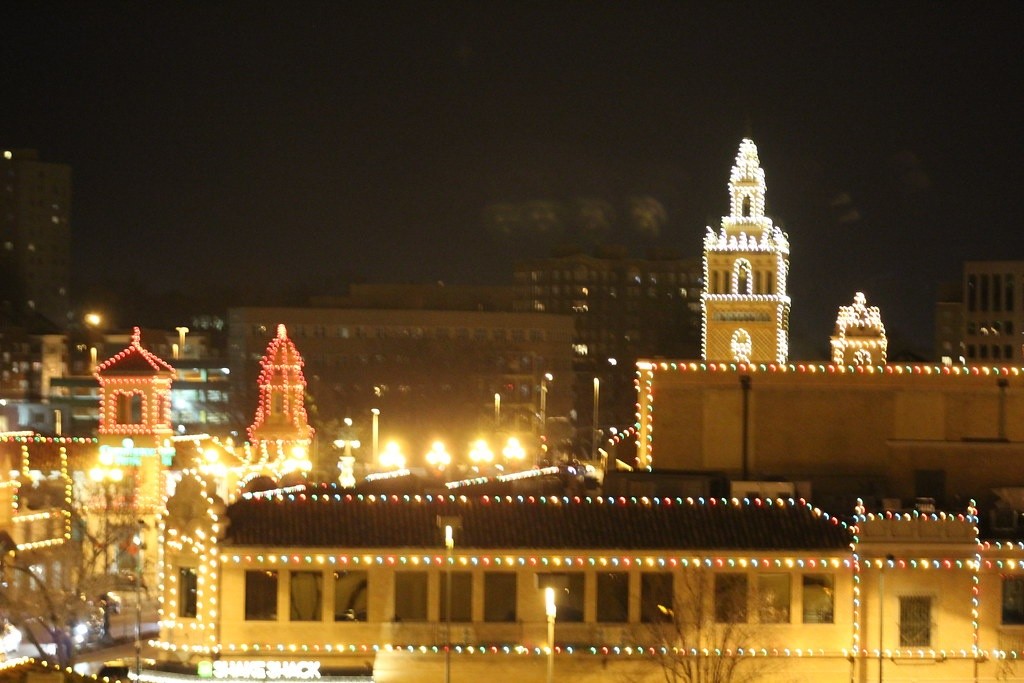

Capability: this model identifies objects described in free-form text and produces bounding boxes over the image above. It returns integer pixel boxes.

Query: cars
[66,593,122,615]
[1,620,23,655]
[49,604,106,646]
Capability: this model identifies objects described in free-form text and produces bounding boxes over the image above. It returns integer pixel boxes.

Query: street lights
[544,585,558,680]
[593,357,617,462]
[540,372,552,434]
[133,526,144,676]
